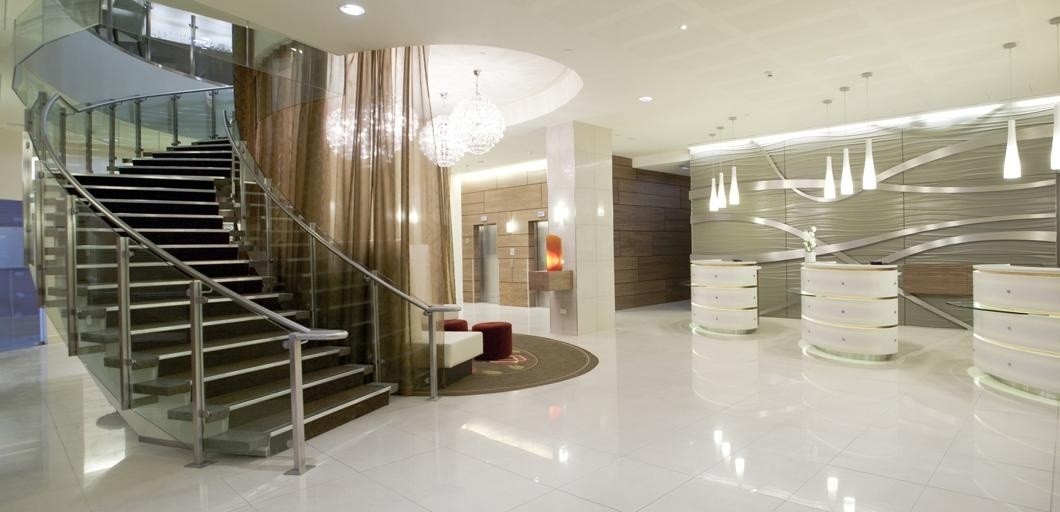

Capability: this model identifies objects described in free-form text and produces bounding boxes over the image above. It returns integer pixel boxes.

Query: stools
[439,319,470,330]
[471,321,513,363]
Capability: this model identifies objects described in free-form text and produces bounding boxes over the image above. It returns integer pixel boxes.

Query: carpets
[391,332,600,396]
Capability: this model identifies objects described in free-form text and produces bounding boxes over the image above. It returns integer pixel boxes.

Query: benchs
[405,330,486,390]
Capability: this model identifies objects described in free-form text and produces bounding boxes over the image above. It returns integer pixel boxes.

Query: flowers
[799,223,819,254]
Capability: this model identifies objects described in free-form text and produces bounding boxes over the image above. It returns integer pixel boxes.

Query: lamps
[419,89,467,170]
[322,76,375,163]
[1001,41,1023,180]
[707,111,741,214]
[860,71,878,192]
[450,70,508,159]
[545,234,564,272]
[1048,16,1060,172]
[823,97,836,202]
[365,70,420,163]
[839,86,854,198]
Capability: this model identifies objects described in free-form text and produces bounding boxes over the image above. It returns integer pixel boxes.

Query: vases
[804,253,817,264]
